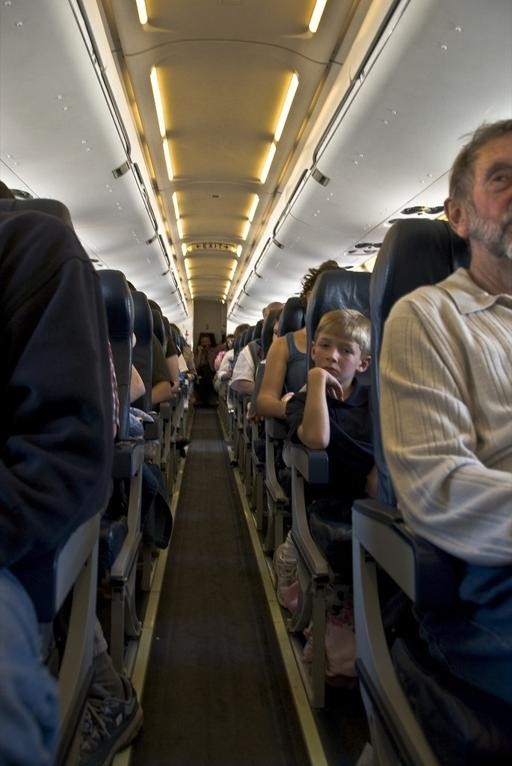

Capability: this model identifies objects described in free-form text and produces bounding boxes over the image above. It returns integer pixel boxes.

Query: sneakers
[302,620,357,677]
[272,544,301,609]
[78,671,146,766]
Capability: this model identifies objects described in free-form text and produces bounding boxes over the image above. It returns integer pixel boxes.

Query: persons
[212,258,384,691]
[377,109,512,708]
[0,182,120,764]
[194,334,214,407]
[76,335,199,766]
